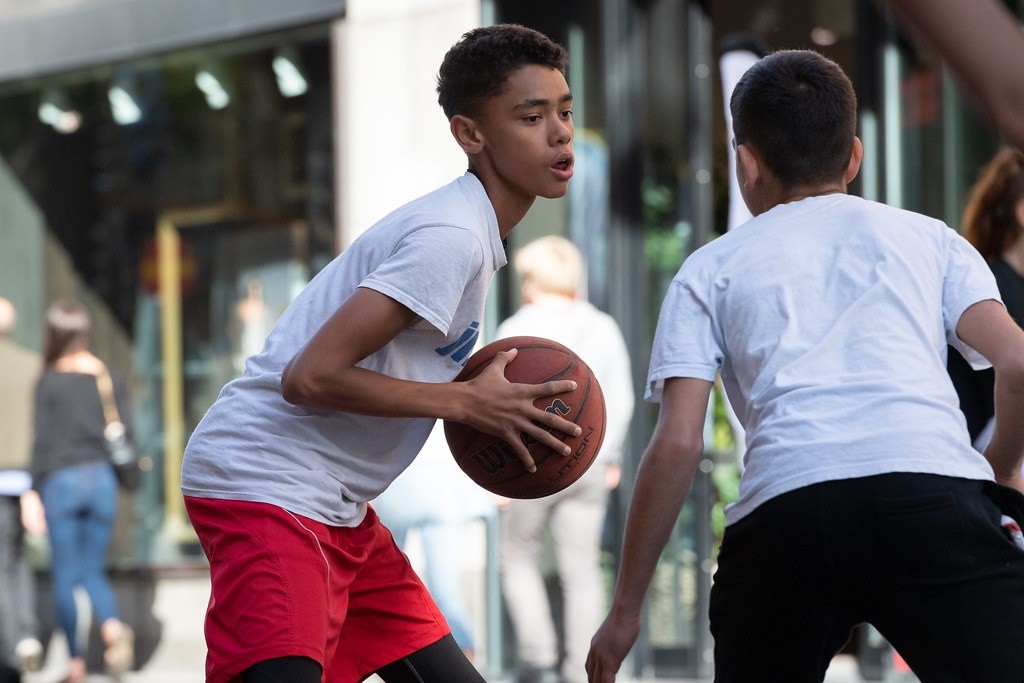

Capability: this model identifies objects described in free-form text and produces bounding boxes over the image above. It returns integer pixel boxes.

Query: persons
[179,24,582,683]
[0,294,140,683]
[494,237,635,683]
[586,49,1024,683]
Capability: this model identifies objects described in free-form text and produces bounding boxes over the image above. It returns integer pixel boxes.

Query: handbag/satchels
[105,430,140,494]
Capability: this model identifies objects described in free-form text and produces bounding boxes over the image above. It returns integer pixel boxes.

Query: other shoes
[101,621,134,672]
[68,659,86,680]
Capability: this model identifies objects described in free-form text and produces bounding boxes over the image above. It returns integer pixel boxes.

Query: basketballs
[443,336,607,500]
